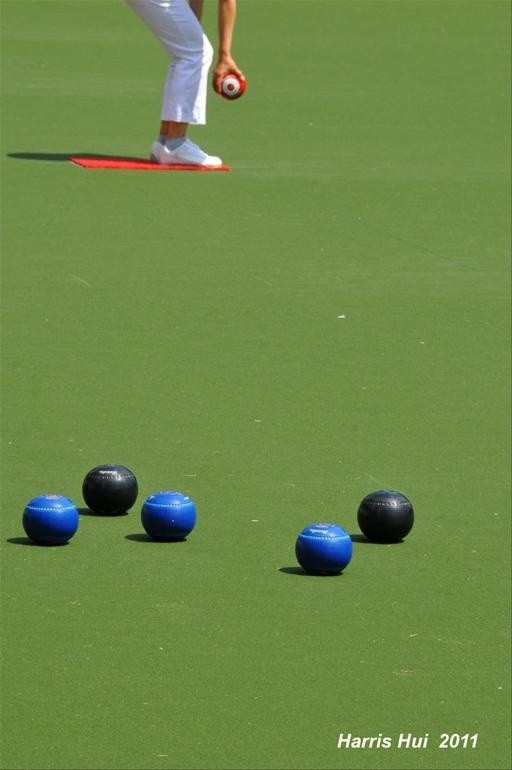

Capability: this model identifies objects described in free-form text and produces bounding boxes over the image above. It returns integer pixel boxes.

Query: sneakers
[151,139,163,163]
[159,139,222,168]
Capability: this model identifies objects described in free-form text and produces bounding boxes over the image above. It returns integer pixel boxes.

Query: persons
[127,0,245,166]
